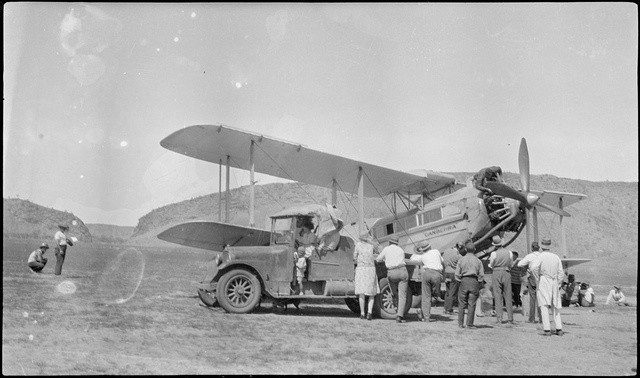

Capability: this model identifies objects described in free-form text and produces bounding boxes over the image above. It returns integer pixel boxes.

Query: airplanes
[157,125,591,307]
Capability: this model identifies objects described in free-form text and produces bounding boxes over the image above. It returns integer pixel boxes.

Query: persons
[54,223,73,277]
[317,220,344,254]
[27,243,49,273]
[488,235,513,326]
[455,243,485,327]
[603,285,631,310]
[376,235,409,322]
[443,242,465,314]
[294,246,307,296]
[517,241,542,322]
[531,238,566,335]
[353,231,381,320]
[296,222,316,258]
[411,242,445,322]
[510,251,526,311]
[560,274,595,308]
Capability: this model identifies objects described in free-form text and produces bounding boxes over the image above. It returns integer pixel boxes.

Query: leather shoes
[396,317,402,323]
[367,312,372,319]
[360,314,365,319]
[538,331,551,336]
[556,330,562,335]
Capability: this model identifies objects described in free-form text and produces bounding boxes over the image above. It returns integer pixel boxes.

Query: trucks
[210,205,446,320]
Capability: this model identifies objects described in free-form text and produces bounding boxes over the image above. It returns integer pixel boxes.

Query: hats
[419,241,430,250]
[359,232,369,239]
[58,223,68,228]
[568,274,575,280]
[491,235,504,246]
[41,243,49,248]
[615,285,621,290]
[539,237,554,249]
[580,282,586,288]
[389,237,398,245]
